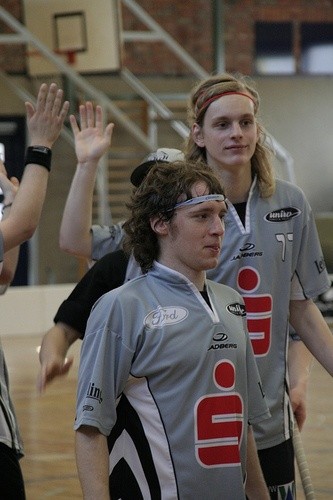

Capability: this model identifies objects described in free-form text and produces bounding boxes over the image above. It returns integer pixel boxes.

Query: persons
[37,100,312,431]
[124,74,333,500]
[0,82,70,500]
[74,158,270,500]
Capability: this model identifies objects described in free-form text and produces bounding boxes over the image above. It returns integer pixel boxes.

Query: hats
[130,148,184,187]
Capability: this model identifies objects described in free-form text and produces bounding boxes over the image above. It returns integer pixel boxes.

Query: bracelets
[23,145,52,171]
